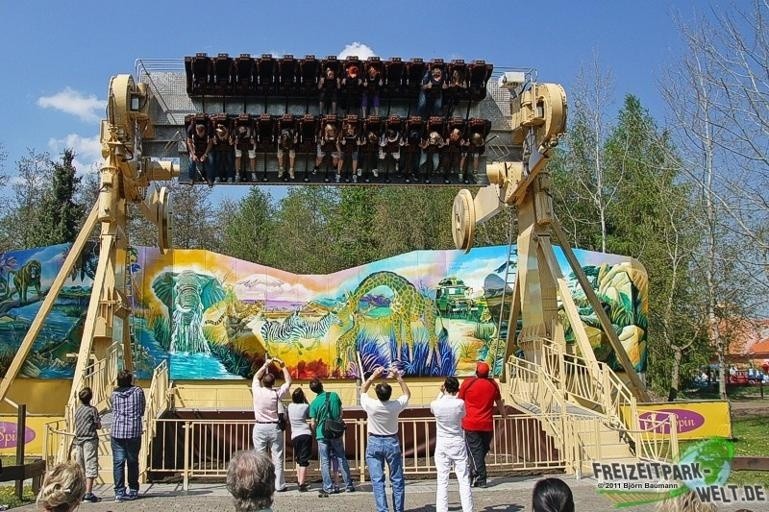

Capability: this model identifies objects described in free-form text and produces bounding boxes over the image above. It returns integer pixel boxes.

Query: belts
[256,422,279,424]
[367,432,398,438]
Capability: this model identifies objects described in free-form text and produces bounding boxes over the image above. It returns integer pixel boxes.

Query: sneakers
[83,493,101,502]
[297,482,312,492]
[128,491,138,499]
[372,169,379,177]
[114,489,130,503]
[471,170,482,184]
[457,170,464,182]
[208,179,213,188]
[335,174,341,183]
[288,169,295,179]
[277,168,286,179]
[352,175,358,183]
[190,179,194,186]
[312,166,320,175]
[357,168,362,176]
[252,173,258,181]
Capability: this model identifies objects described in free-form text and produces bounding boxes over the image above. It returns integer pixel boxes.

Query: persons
[251,357,292,493]
[186,64,485,185]
[359,366,411,512]
[309,377,355,494]
[35,460,87,512]
[531,477,575,512]
[73,386,102,503]
[287,387,312,492]
[109,369,146,503]
[457,361,507,488]
[226,449,276,512]
[430,376,475,512]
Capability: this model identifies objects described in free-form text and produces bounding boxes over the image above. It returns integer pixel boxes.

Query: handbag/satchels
[323,418,348,439]
[277,398,288,432]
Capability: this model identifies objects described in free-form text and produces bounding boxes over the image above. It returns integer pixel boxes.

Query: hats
[476,362,489,374]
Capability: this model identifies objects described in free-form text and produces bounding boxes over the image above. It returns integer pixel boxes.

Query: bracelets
[261,363,268,370]
[398,378,405,384]
[369,376,375,387]
